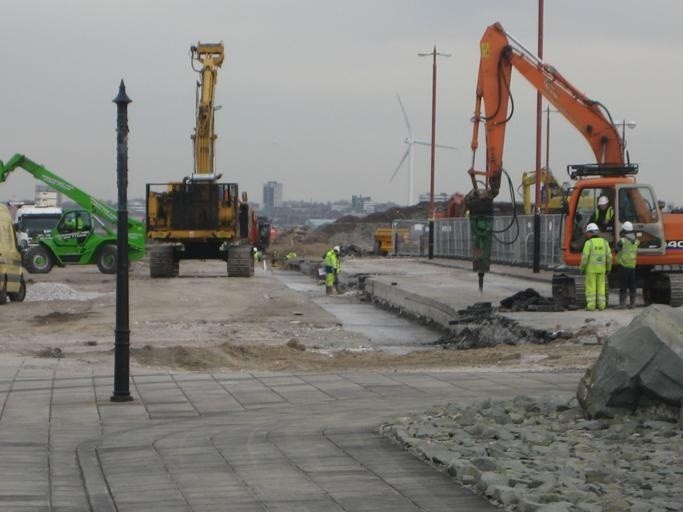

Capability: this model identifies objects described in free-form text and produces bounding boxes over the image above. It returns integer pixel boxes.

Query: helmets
[622,221,633,231]
[597,195,609,206]
[586,222,599,232]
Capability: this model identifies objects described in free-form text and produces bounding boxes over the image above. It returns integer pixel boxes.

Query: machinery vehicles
[516,165,597,215]
[464,19,682,309]
[146,39,273,279]
[0,150,147,275]
[371,193,464,255]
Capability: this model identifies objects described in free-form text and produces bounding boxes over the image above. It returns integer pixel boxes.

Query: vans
[0,203,29,304]
[11,203,64,254]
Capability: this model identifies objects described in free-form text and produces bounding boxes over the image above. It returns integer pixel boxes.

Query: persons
[64,212,84,232]
[578,222,612,311]
[320,245,347,296]
[271,249,297,267]
[611,221,641,309]
[585,195,615,233]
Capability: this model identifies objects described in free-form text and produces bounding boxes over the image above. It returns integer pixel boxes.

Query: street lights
[417,45,453,218]
[614,116,638,141]
